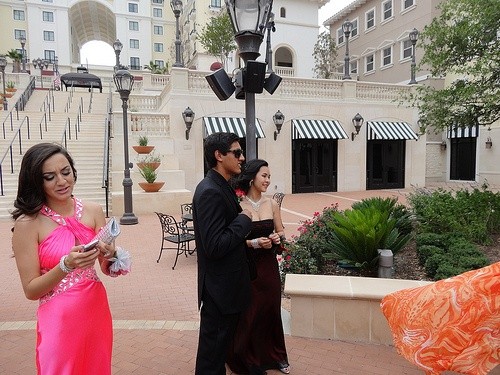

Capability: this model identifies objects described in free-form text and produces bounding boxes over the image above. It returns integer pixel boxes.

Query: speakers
[245,59,266,93]
[265,72,282,94]
[205,68,236,101]
[233,68,245,99]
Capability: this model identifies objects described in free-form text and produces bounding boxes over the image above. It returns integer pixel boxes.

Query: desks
[183,215,196,255]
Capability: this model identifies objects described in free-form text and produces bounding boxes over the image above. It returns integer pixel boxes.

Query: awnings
[203,117,266,140]
[291,120,349,140]
[367,121,419,140]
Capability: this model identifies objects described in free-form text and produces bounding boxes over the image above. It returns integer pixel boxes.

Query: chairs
[181,204,195,255]
[155,212,196,269]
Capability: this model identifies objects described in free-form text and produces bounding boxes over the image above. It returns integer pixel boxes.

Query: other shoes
[280,365,290,373]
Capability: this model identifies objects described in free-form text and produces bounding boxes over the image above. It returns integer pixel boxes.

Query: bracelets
[60,255,76,274]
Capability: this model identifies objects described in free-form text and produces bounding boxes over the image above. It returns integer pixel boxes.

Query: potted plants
[133,137,154,154]
[0,99,5,104]
[138,164,165,191]
[137,159,160,172]
[5,81,17,93]
[0,92,14,97]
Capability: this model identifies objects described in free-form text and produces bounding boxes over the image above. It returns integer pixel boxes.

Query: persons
[192,132,253,375]
[234,159,291,375]
[11,142,116,375]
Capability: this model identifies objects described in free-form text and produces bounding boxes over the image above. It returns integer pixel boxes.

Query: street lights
[204,0,284,164]
[265,11,275,74]
[113,65,139,226]
[18,34,28,73]
[341,18,353,80]
[408,28,419,84]
[112,38,123,69]
[0,56,8,110]
[169,0,184,68]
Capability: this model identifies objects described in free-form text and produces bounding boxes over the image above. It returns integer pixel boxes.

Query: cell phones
[83,239,99,252]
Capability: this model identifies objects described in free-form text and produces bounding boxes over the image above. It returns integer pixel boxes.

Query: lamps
[486,137,492,148]
[441,140,447,151]
[273,110,284,141]
[182,107,195,140]
[351,113,363,141]
[415,116,425,136]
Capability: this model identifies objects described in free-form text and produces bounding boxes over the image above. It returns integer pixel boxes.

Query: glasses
[226,148,243,157]
